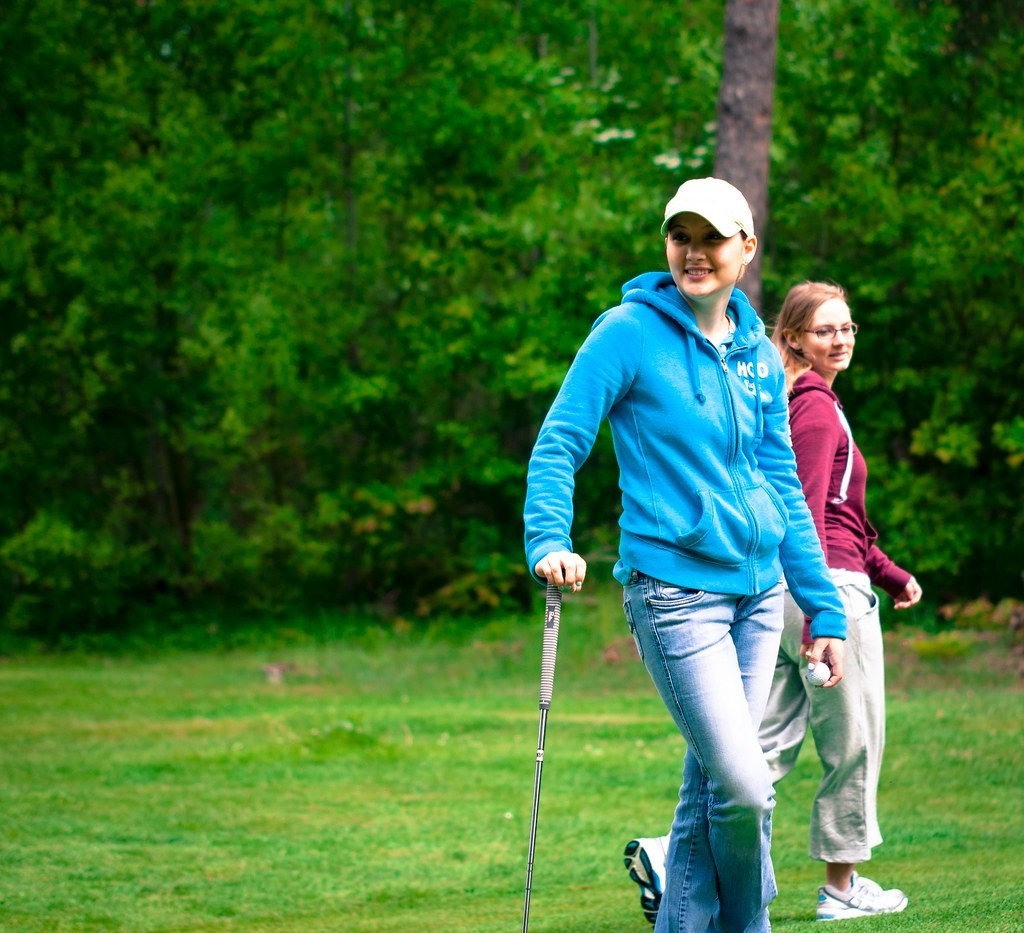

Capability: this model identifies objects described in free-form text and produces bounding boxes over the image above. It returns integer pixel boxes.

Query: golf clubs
[522,579,564,932]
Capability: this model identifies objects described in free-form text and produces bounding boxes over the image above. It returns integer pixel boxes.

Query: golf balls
[805,662,831,687]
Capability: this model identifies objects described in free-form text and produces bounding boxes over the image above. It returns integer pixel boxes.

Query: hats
[661,178,754,239]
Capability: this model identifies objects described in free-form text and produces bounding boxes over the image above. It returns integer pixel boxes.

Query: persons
[624,284,923,929]
[523,177,847,933]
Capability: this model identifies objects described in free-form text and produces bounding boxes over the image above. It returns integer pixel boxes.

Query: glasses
[797,323,858,339]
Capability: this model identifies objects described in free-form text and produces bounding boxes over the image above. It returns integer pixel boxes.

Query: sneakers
[816,871,908,920]
[623,835,668,928]
